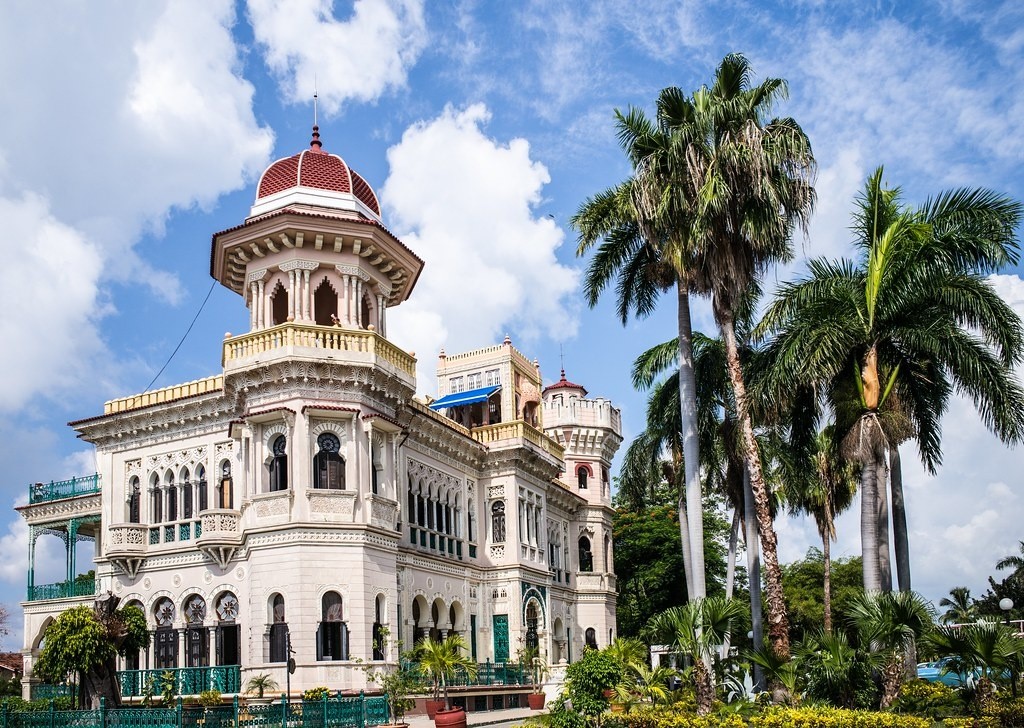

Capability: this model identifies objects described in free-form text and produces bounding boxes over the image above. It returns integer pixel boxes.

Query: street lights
[747,630,756,685]
[999,597,1018,700]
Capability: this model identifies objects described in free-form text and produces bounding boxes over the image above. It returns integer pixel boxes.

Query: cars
[916,655,1010,689]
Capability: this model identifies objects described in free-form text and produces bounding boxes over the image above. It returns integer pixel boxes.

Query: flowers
[303,686,330,701]
[197,688,223,707]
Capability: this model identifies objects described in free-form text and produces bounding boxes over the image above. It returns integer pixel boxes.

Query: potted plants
[507,644,551,710]
[399,633,480,728]
